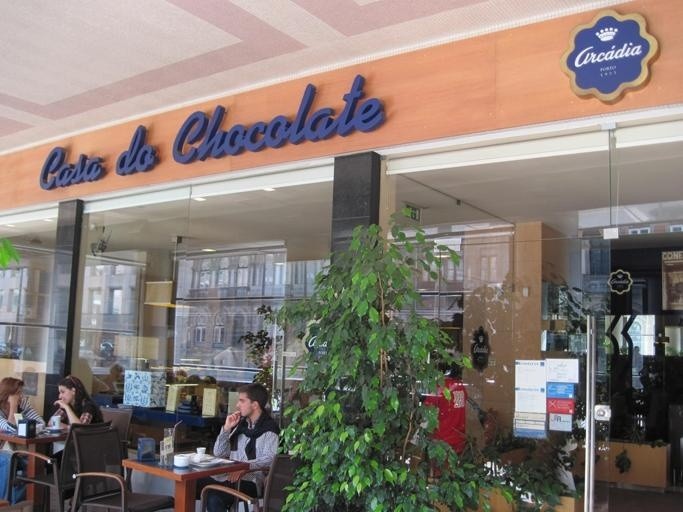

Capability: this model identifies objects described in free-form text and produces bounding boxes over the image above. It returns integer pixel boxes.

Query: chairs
[7,406,305,512]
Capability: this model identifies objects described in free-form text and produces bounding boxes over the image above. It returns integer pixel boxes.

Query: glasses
[65,374,75,384]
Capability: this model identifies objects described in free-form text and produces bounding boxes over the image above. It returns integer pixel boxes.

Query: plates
[196,459,219,468]
[46,426,65,432]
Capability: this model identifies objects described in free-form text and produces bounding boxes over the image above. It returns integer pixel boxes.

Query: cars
[100,342,115,349]
[0,344,22,359]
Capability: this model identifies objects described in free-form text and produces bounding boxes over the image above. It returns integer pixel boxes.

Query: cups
[51,416,61,428]
[191,447,206,464]
[159,428,175,468]
[173,454,189,468]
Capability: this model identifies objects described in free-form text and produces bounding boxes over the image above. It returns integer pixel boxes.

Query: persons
[93,362,226,414]
[195,382,281,512]
[47,375,104,475]
[420,354,468,481]
[0,377,46,506]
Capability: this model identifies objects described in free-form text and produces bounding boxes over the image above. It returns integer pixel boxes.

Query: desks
[0,424,70,512]
